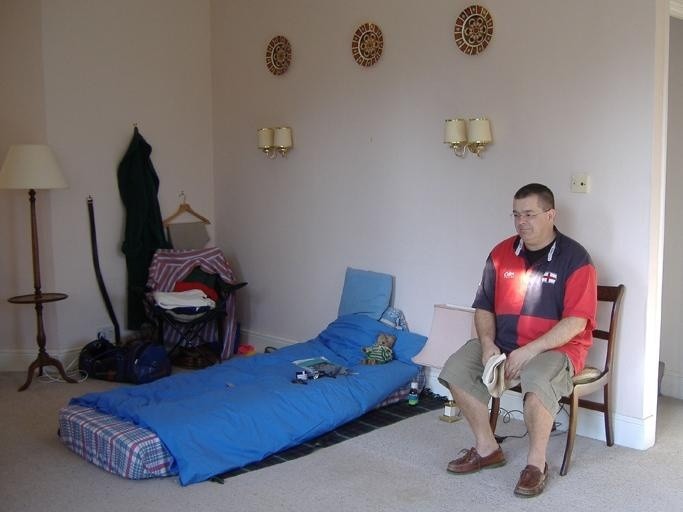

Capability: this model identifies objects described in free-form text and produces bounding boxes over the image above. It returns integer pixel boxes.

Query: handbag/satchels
[79,331,171,385]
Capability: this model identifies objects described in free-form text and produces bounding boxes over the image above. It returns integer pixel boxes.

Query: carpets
[208,382,451,484]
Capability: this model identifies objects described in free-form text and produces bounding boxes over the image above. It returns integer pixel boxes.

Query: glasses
[511,209,551,221]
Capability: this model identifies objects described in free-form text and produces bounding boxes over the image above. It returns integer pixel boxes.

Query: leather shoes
[166,353,194,370]
[446,445,506,476]
[514,463,549,497]
[195,354,218,369]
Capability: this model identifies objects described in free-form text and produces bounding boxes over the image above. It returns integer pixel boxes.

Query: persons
[438,183,598,496]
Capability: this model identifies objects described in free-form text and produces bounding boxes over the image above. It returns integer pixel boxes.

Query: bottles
[407,380,420,406]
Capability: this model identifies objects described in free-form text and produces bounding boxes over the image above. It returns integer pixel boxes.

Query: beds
[57,315,427,484]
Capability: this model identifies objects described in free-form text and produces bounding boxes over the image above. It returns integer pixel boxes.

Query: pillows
[387,330,428,366]
[319,311,395,363]
[338,265,394,328]
[173,281,219,303]
[378,306,407,332]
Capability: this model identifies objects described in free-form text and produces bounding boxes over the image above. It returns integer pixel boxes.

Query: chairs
[490,282,624,475]
[149,247,247,357]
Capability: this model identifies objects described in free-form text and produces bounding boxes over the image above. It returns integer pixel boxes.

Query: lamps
[411,302,484,421]
[443,117,493,161]
[0,143,79,393]
[256,126,294,156]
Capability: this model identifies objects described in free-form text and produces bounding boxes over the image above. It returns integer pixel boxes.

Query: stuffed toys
[361,333,396,366]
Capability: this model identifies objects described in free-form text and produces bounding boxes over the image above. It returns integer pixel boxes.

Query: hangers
[160,191,212,227]
